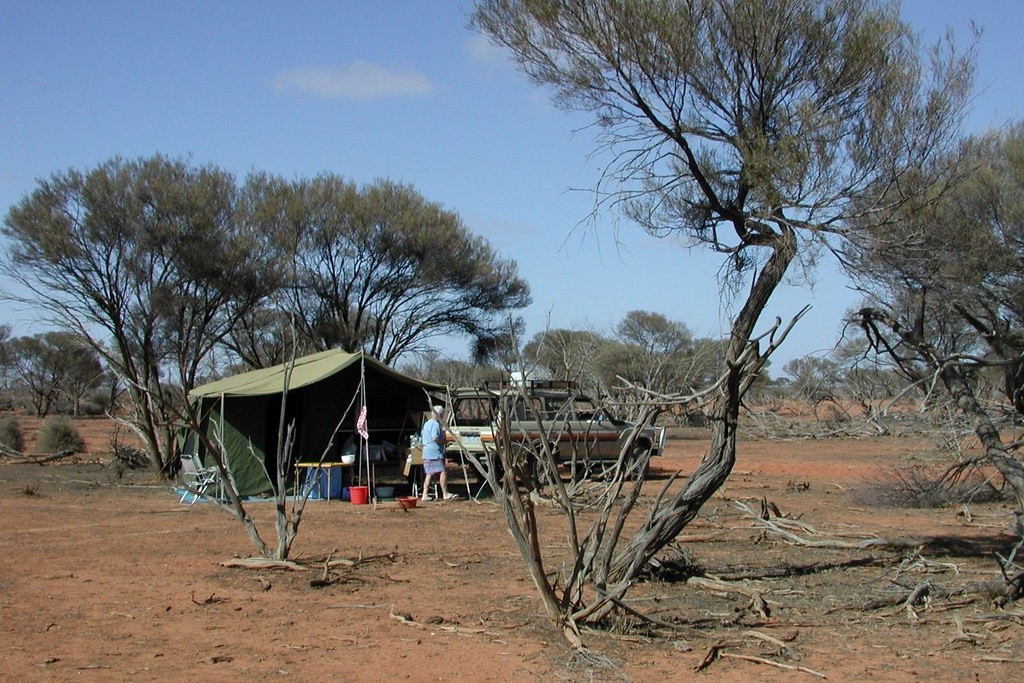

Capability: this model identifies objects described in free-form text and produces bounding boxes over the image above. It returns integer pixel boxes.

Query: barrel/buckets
[350,486,368,504]
[510,371,535,387]
[341,487,350,501]
[301,467,342,498]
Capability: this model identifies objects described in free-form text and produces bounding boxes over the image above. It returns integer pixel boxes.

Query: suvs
[443,378,668,486]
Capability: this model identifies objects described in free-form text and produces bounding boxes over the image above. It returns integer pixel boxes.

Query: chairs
[179,452,225,507]
[361,444,396,480]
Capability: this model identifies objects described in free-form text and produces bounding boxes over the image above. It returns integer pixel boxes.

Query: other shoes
[421,498,437,501]
[443,494,458,500]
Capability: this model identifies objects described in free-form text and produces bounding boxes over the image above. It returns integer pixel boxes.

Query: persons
[421,405,459,501]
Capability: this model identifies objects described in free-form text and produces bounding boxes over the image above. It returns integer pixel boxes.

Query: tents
[181,347,470,503]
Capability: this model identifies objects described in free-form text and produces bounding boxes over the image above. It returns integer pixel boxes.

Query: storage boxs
[403,446,422,476]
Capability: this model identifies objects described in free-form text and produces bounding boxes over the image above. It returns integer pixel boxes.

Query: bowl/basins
[395,496,418,508]
[341,455,355,463]
[376,487,394,497]
[377,498,395,503]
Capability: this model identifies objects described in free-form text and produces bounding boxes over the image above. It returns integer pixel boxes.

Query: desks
[412,465,439,500]
[294,462,356,505]
